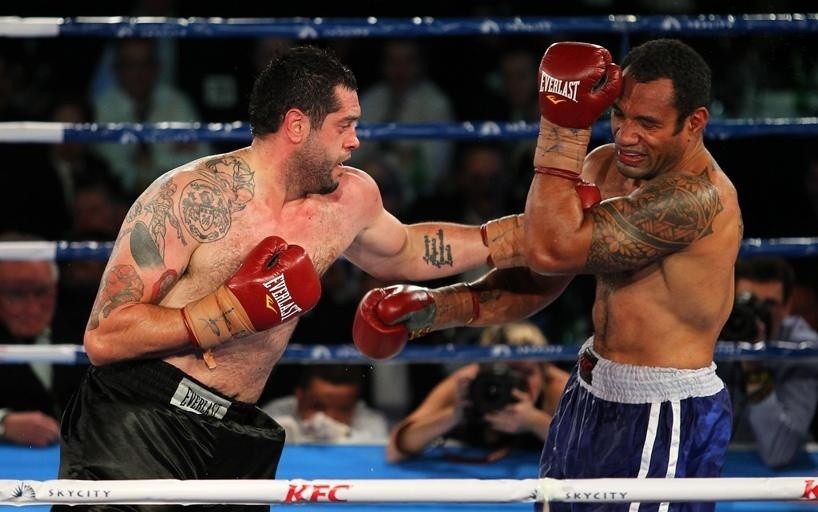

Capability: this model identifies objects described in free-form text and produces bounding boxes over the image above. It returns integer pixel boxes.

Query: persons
[353,37,744,512]
[50,45,603,512]
[1,0,817,467]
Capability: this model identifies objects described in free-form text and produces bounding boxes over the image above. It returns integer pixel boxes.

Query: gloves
[178,237,323,368]
[482,184,603,271]
[532,41,626,181]
[351,284,481,361]
